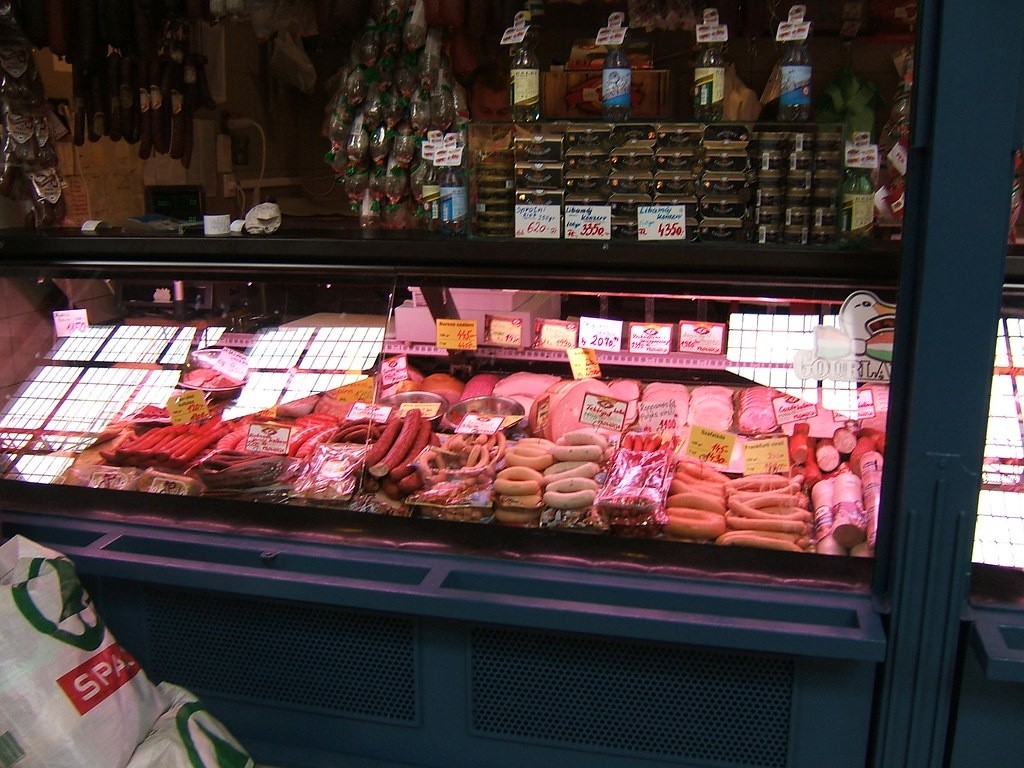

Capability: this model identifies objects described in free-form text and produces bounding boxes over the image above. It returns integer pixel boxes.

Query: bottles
[600,11,630,119]
[837,131,877,248]
[691,6,725,121]
[780,5,813,122]
[420,131,445,233]
[509,10,539,124]
[437,131,470,237]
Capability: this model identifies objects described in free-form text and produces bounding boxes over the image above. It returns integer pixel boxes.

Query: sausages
[100,410,883,558]
[0,1,195,232]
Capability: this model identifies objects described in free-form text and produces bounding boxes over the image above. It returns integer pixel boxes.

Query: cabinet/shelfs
[1,227,898,590]
[465,120,843,247]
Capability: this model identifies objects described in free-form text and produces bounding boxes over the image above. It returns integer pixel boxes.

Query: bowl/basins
[176,382,246,401]
[444,396,526,438]
[378,390,448,432]
